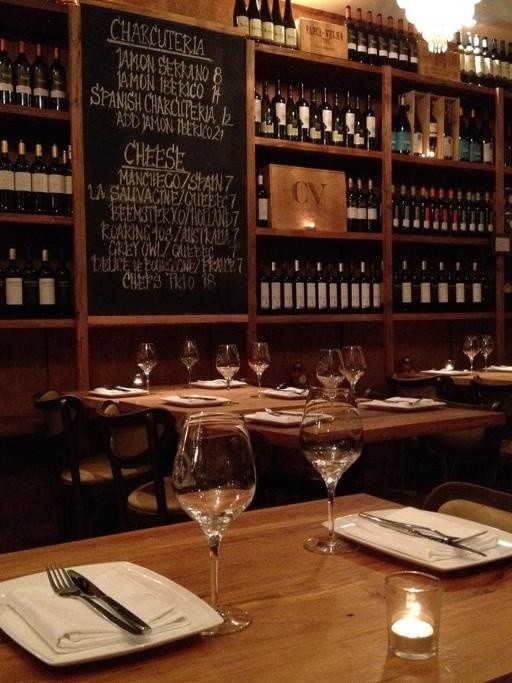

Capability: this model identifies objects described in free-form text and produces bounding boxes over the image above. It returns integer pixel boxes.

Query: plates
[261,386,310,399]
[367,394,448,413]
[242,408,335,426]
[484,366,512,372]
[320,506,512,575]
[0,562,225,668]
[87,386,147,398]
[420,368,473,376]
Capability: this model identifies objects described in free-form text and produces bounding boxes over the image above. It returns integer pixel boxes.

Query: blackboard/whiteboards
[80,2,249,316]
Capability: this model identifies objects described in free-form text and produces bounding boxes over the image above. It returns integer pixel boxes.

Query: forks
[358,512,488,543]
[263,407,322,418]
[380,397,424,406]
[42,563,144,636]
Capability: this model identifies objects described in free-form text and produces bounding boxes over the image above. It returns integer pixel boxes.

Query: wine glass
[132,339,271,408]
[170,410,256,639]
[298,387,365,555]
[316,345,366,408]
[463,334,495,375]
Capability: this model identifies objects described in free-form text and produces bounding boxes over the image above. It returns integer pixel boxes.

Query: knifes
[367,519,486,557]
[66,569,152,631]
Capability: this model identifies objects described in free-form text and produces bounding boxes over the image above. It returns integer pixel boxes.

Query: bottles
[346,176,494,239]
[0,37,73,320]
[251,74,512,170]
[258,255,491,314]
[342,6,421,76]
[290,361,308,387]
[233,0,300,50]
[255,173,269,227]
[400,357,414,379]
[456,30,511,92]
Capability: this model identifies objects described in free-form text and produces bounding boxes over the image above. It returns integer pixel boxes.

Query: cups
[383,570,443,662]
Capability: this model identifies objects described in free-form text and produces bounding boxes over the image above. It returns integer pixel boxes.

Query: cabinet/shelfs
[0,0,81,332]
[246,33,512,324]
